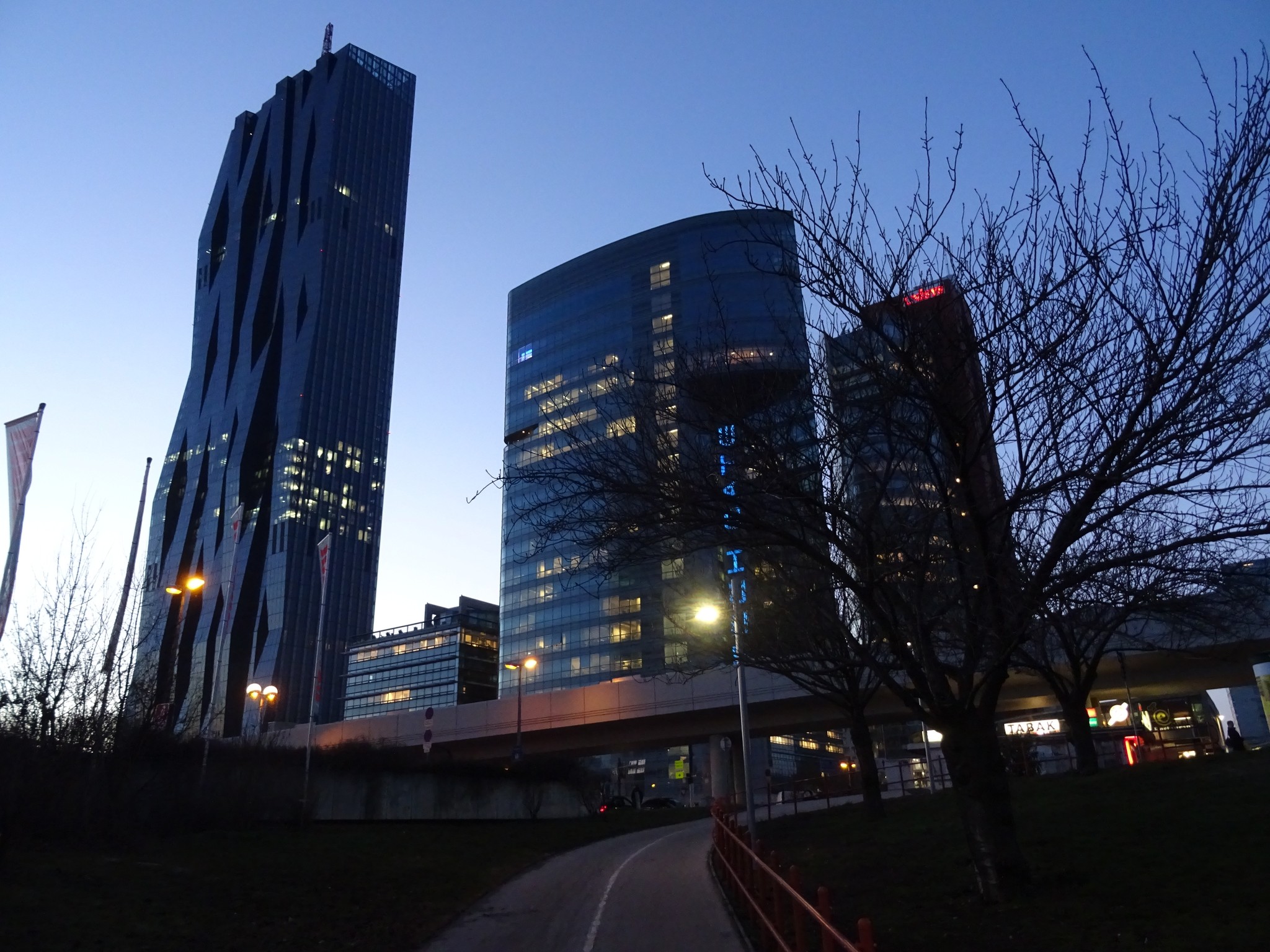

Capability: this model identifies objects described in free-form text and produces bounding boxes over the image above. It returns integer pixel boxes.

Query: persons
[1227,721,1246,752]
[631,783,640,802]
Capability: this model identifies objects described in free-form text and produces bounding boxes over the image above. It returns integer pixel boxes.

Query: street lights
[1116,650,1143,764]
[691,574,760,857]
[166,573,207,703]
[245,683,279,746]
[504,654,539,754]
